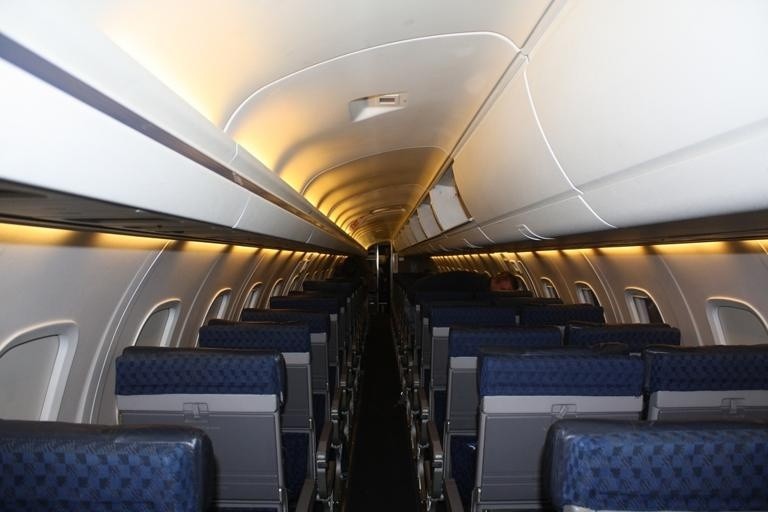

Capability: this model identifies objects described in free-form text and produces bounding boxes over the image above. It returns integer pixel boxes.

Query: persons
[491,273,520,291]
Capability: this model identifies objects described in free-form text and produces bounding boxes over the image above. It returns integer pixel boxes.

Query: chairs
[1,276,366,512]
[392,271,762,508]
